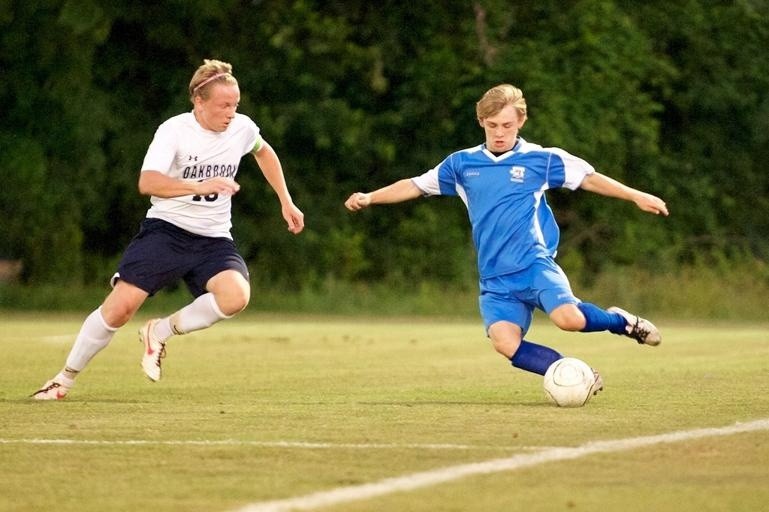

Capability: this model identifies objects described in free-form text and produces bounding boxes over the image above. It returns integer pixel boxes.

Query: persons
[28,57,306,405]
[342,84,670,399]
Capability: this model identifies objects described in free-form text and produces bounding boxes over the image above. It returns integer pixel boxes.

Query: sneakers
[589,367,603,400]
[138,318,167,382]
[606,306,662,346]
[30,376,72,400]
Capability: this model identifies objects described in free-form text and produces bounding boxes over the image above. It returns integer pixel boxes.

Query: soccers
[543,357,596,408]
